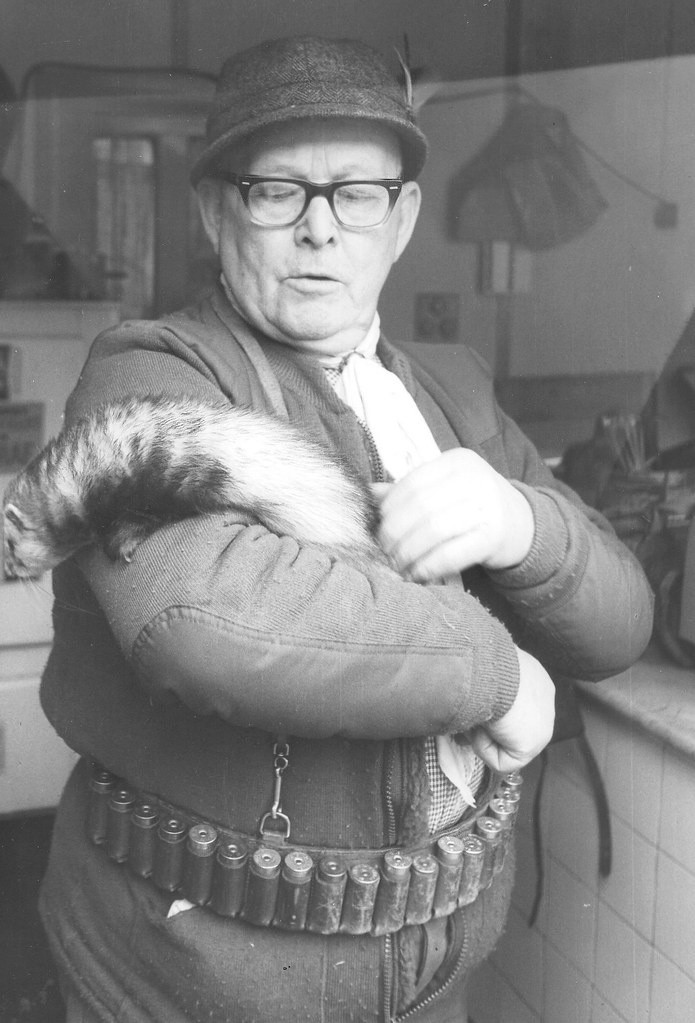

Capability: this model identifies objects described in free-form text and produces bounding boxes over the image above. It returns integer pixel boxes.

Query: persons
[37,37,656,1023]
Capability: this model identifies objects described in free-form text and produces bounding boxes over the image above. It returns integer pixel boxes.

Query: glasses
[190,161,408,229]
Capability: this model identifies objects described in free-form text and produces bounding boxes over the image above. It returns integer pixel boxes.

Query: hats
[193,34,429,183]
